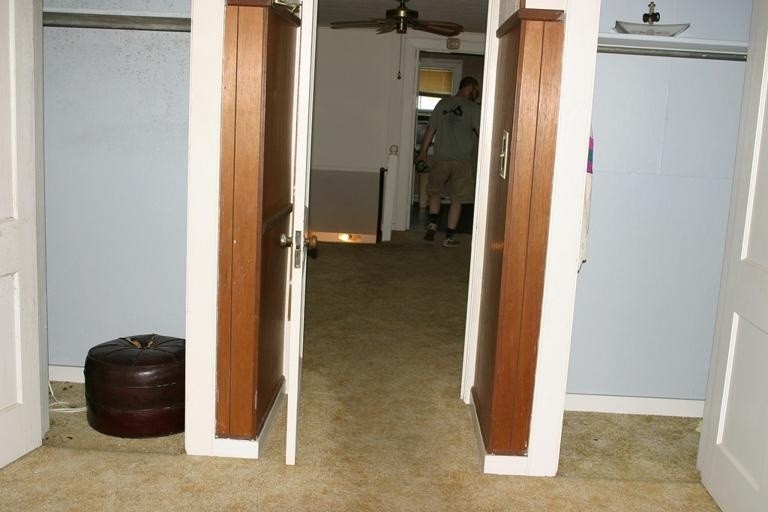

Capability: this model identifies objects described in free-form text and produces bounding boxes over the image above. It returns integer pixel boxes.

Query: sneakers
[424,221,438,241]
[442,237,463,248]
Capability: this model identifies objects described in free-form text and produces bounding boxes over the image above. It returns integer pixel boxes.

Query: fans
[330,1,465,38]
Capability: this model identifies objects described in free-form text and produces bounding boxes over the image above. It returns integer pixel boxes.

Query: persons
[414,76,480,247]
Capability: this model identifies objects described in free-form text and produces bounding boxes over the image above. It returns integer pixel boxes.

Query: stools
[82,332,185,439]
